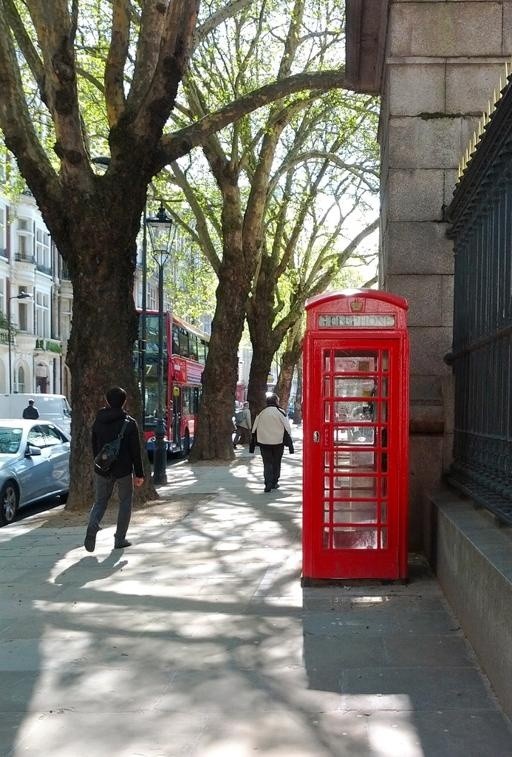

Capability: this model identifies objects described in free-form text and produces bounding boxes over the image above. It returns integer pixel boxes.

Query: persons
[233,401,252,450]
[249,392,294,493]
[23,398,39,418]
[84,386,146,552]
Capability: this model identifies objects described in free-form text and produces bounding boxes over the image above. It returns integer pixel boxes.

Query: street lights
[145,196,183,486]
[7,288,34,395]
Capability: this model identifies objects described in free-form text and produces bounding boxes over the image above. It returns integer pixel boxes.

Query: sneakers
[84,527,98,552]
[114,537,132,549]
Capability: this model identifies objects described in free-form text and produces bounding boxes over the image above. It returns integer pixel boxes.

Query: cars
[230,380,297,422]
[0,416,72,528]
[0,390,72,440]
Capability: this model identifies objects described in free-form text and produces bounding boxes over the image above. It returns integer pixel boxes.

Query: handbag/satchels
[94,432,125,473]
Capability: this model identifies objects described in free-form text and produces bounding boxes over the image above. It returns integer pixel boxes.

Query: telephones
[369,369,387,422]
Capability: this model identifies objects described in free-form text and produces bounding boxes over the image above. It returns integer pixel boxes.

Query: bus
[133,305,212,461]
